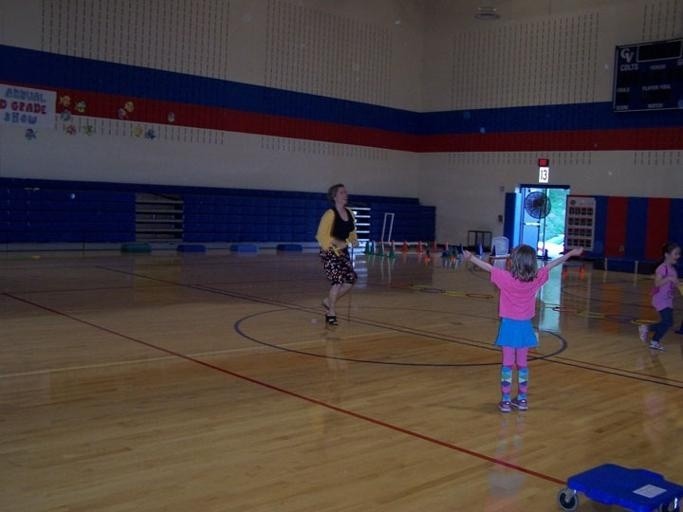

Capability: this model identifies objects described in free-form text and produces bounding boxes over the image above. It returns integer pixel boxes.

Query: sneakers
[638,324,649,343]
[511,397,528,410]
[649,341,666,351]
[497,400,512,413]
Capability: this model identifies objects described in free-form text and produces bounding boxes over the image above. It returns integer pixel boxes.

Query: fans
[525,192,551,259]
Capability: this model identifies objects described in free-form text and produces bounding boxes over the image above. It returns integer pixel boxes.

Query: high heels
[322,302,339,326]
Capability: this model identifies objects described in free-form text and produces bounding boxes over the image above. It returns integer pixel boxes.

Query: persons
[643,351,682,466]
[311,329,350,439]
[312,182,359,330]
[460,242,585,414]
[487,415,527,511]
[638,242,682,351]
